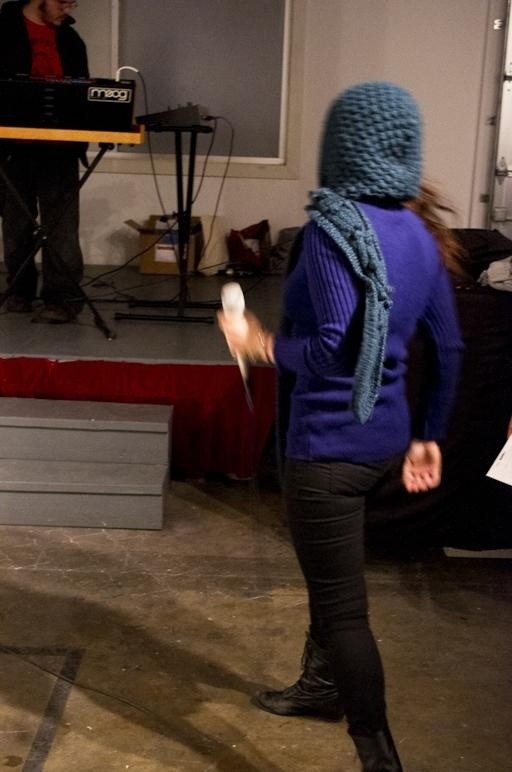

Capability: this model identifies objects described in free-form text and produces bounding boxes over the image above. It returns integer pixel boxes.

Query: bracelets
[259,329,276,368]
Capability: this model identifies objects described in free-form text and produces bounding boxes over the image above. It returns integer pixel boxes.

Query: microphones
[220,282,257,410]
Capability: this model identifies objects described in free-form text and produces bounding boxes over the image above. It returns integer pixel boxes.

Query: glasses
[60,2,80,9]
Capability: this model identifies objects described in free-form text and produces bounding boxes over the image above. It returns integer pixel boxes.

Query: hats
[320,79,421,201]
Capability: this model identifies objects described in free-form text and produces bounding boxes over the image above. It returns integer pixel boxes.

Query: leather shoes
[6,294,83,323]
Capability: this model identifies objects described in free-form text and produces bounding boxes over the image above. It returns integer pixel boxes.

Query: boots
[347,728,403,772]
[256,630,345,721]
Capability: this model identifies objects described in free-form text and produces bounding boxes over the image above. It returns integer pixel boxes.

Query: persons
[0,0,88,324]
[215,84,464,772]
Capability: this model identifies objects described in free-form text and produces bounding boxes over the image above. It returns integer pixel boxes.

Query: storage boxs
[120,209,207,280]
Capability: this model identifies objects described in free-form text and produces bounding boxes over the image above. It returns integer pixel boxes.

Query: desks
[0,117,149,339]
